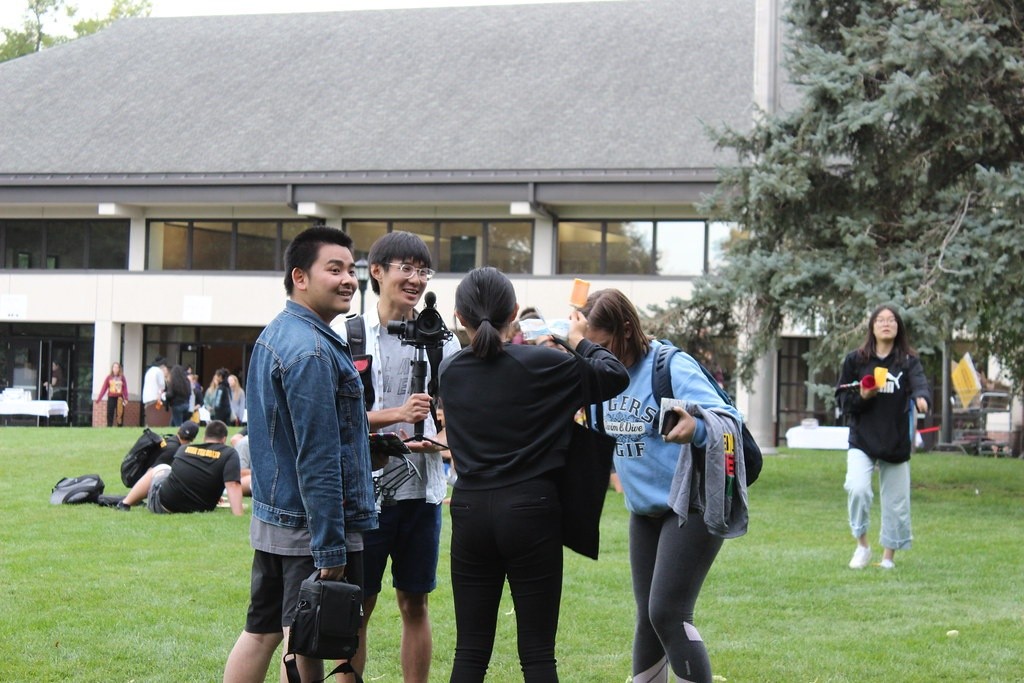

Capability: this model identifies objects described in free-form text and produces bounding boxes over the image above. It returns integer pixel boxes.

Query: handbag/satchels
[190,410,200,423]
[283,569,364,683]
[553,338,617,562]
[156,400,161,409]
[98,494,146,506]
[242,409,248,422]
[198,405,211,420]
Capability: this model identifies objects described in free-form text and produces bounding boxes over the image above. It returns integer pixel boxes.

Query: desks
[0,400,69,428]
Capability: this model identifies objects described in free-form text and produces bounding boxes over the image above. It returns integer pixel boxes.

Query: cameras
[386,309,452,349]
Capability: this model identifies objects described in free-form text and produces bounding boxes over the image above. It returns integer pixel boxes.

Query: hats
[153,356,166,365]
[181,421,199,436]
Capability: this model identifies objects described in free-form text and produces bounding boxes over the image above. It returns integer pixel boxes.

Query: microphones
[424,292,436,307]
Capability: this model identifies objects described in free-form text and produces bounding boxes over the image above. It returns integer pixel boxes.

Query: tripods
[400,342,451,451]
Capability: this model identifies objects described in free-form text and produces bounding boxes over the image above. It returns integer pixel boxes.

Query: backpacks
[652,339,762,486]
[50,474,105,504]
[121,429,174,487]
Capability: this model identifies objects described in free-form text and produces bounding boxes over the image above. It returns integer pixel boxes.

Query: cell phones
[660,410,681,436]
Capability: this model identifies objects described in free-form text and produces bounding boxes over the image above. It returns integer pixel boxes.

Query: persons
[113,418,252,516]
[575,288,764,683]
[331,231,461,683]
[835,304,933,571]
[433,266,631,683]
[42,360,65,401]
[94,357,247,427]
[221,226,380,683]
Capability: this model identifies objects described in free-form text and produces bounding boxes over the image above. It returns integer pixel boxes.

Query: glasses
[385,262,436,283]
[876,317,896,327]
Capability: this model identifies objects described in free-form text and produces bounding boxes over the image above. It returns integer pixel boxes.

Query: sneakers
[881,559,894,567]
[848,544,873,568]
[116,501,130,511]
